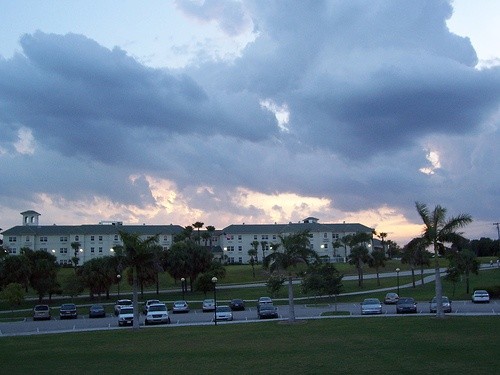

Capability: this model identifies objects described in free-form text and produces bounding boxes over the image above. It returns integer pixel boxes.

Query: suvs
[144,304,170,325]
[114,299,134,315]
[116,307,137,327]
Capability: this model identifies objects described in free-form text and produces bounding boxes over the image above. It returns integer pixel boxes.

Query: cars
[203,298,217,312]
[385,293,399,305]
[32,304,52,321]
[471,290,489,303]
[257,296,273,309]
[429,295,451,314]
[359,297,384,316]
[58,303,77,320]
[145,300,160,313]
[397,297,419,315]
[172,300,189,314]
[212,305,233,322]
[88,303,107,319]
[231,298,244,311]
[258,304,278,320]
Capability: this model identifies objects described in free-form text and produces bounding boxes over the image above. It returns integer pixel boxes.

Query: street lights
[211,276,218,328]
[396,268,401,299]
[180,278,186,299]
[116,274,122,301]
